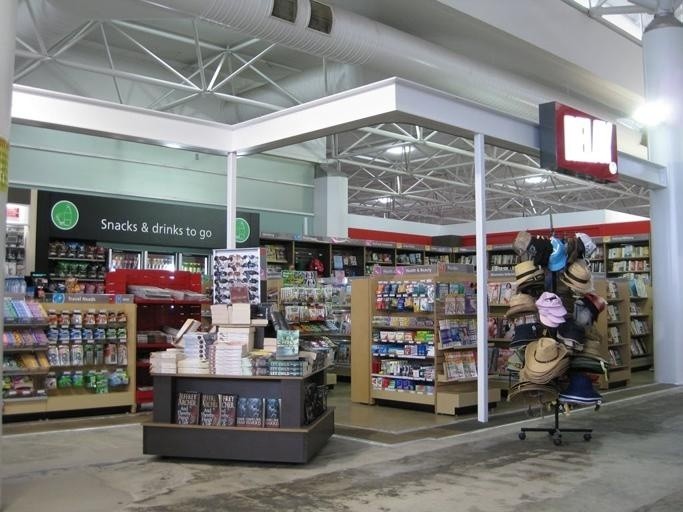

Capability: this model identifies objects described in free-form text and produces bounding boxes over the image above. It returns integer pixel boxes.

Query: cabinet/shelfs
[48,238,107,291]
[4,202,25,278]
[350,275,655,415]
[278,287,337,390]
[108,268,208,405]
[455,239,651,274]
[140,324,337,465]
[1,302,134,419]
[260,238,454,277]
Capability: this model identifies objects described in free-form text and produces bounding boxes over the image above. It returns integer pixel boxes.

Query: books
[149,304,334,428]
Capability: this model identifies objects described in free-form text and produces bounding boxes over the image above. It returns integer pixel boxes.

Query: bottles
[5,245,23,295]
[111,254,204,273]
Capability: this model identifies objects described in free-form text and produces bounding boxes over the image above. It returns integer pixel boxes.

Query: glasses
[213,255,257,301]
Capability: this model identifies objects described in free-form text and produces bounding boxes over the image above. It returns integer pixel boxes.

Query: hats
[505,230,608,406]
